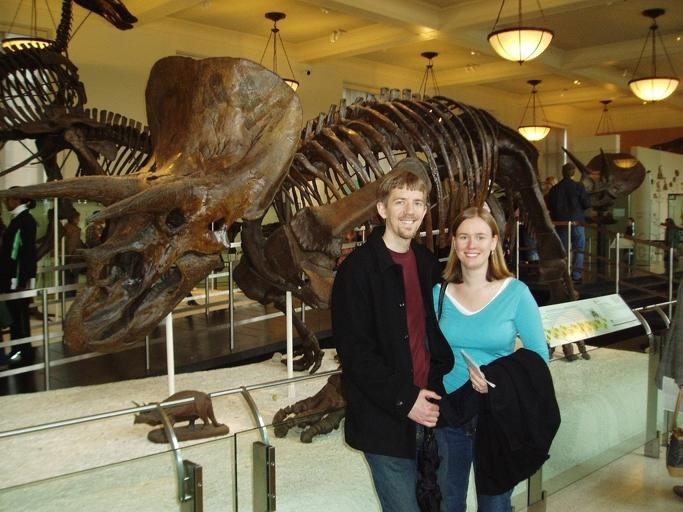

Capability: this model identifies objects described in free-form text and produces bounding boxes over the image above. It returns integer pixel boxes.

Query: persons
[0,186,110,366]
[430,208,549,512]
[331,171,455,512]
[495,163,591,293]
[663,217,680,274]
[626,217,635,265]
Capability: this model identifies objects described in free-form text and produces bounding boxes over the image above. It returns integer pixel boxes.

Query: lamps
[516,77,550,142]
[625,6,681,107]
[487,2,556,70]
